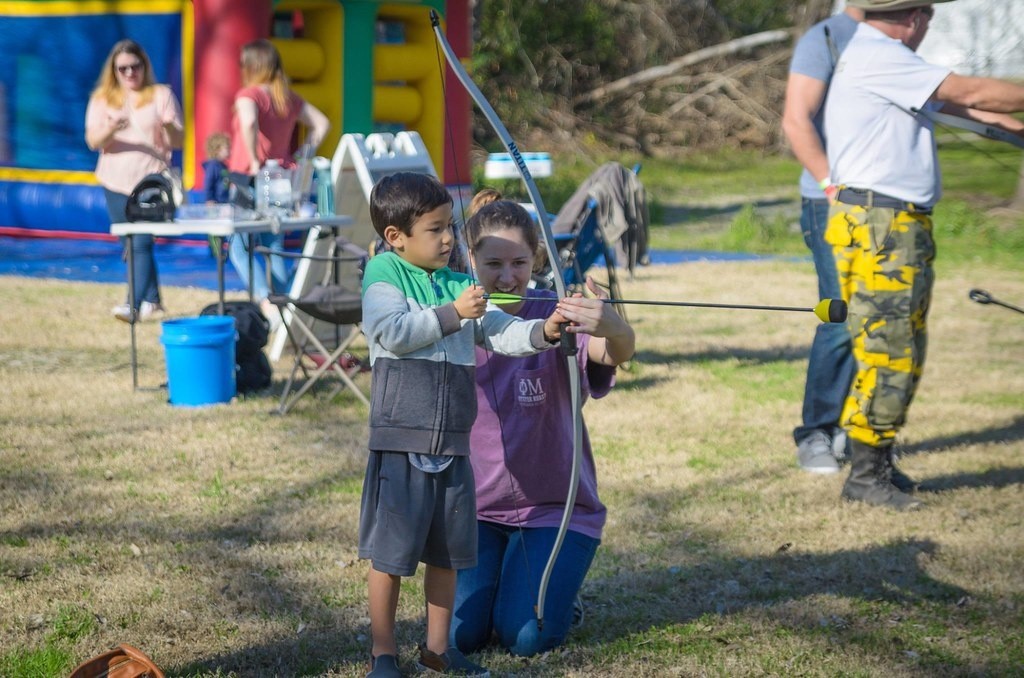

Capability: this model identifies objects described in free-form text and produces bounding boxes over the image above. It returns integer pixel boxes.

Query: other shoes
[114,304,140,323]
[140,302,162,323]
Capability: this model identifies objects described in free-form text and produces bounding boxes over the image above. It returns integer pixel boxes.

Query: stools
[252,238,373,416]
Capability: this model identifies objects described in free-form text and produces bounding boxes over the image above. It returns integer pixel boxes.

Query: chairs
[525,162,645,370]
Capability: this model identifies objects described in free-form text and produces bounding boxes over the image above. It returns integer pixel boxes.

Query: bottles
[256,158,295,220]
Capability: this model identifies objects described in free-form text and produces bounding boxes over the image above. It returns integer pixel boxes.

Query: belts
[836,186,934,214]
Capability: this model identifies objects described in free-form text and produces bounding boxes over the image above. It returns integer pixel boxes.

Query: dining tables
[110,215,354,393]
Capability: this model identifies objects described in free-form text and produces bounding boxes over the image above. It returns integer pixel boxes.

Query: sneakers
[840,440,925,514]
[831,429,858,460]
[364,651,401,678]
[417,644,489,678]
[798,430,840,475]
[871,447,920,493]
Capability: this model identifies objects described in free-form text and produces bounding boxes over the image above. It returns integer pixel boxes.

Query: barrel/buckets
[159,313,242,407]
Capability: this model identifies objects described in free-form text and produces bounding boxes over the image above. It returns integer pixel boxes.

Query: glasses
[114,60,147,74]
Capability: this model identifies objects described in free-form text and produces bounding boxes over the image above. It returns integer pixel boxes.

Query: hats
[845,0,956,12]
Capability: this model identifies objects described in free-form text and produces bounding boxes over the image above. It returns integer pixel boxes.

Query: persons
[781,1,1023,512]
[360,172,636,677]
[86,39,184,322]
[201,132,232,260]
[231,38,332,336]
